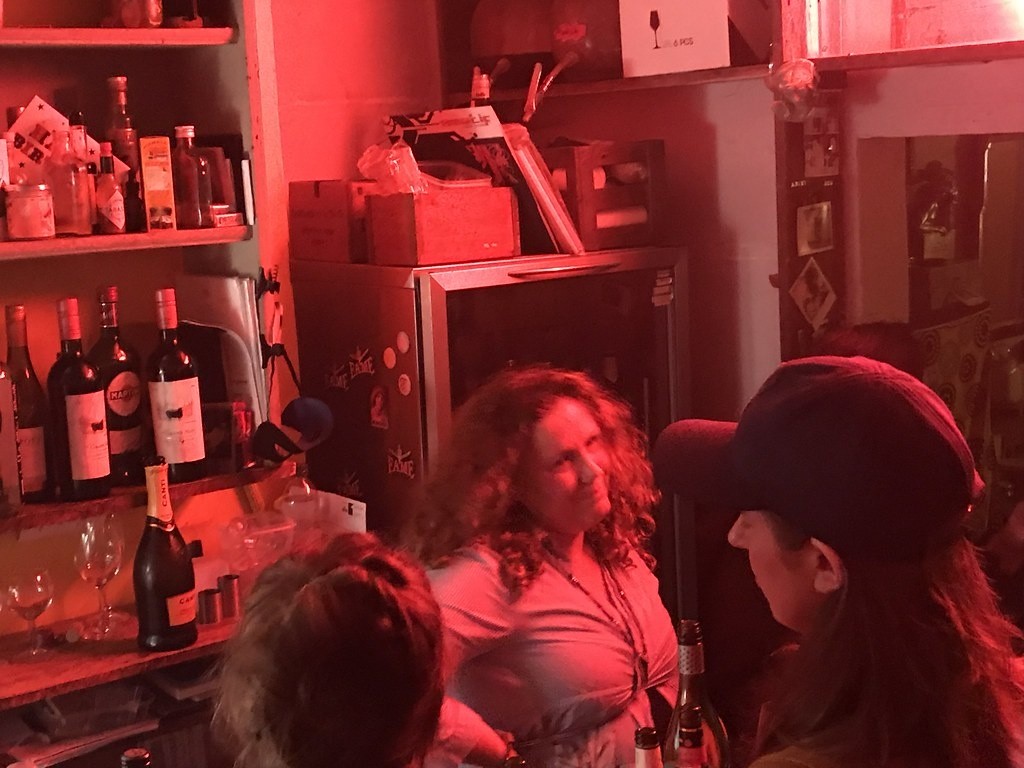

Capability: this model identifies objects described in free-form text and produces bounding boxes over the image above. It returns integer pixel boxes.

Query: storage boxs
[618,1,775,77]
[291,138,670,267]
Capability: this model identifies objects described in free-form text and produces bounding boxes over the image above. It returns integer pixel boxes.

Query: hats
[653,355,976,560]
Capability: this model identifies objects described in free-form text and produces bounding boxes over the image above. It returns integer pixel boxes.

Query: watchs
[505,756,527,768]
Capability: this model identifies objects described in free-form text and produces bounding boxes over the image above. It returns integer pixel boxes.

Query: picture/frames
[789,107,839,334]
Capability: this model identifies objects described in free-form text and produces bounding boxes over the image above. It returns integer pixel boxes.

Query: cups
[5,181,55,239]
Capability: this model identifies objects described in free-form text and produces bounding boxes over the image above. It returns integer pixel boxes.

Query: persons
[651,356,1024,768]
[808,137,839,168]
[212,533,443,768]
[419,370,728,768]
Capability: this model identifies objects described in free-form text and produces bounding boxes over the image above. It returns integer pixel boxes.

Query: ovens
[293,243,695,668]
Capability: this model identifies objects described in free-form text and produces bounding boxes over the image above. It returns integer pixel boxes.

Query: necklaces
[566,560,649,686]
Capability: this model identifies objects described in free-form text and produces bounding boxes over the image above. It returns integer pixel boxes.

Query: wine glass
[5,569,55,661]
[68,511,125,644]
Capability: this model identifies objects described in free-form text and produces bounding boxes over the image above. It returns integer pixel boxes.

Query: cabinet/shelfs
[0,0,281,537]
[0,589,250,768]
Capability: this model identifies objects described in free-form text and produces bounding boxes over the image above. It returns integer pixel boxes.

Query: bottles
[170,124,214,229]
[632,725,664,767]
[470,72,493,111]
[44,131,93,235]
[87,284,148,487]
[672,701,711,768]
[104,74,141,199]
[7,105,26,131]
[281,464,318,527]
[46,294,110,501]
[129,455,199,654]
[665,617,730,768]
[120,747,153,768]
[69,109,87,158]
[4,303,48,501]
[97,142,126,235]
[146,287,210,482]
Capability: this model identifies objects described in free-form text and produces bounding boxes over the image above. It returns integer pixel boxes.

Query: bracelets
[495,730,514,768]
